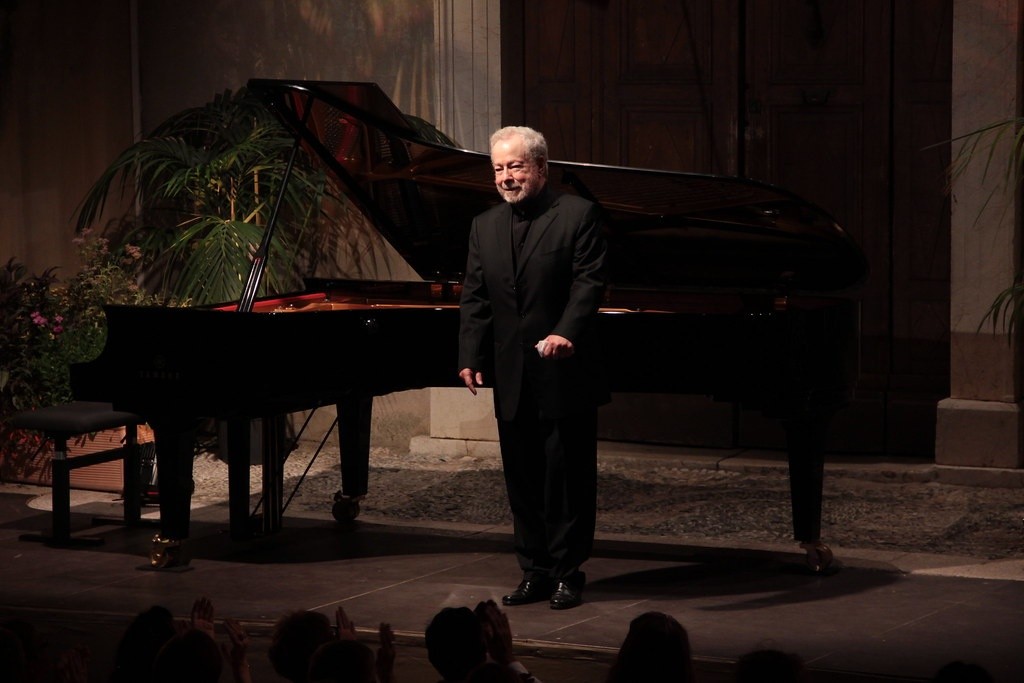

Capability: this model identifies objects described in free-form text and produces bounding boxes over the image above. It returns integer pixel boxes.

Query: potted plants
[65,85,457,465]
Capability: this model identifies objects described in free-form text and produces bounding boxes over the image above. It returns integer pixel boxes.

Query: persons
[1,595,1002,683]
[454,123,611,610]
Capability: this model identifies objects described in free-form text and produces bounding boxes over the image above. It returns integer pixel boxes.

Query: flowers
[1,227,194,490]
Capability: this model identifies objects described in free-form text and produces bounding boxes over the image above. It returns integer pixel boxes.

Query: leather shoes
[551,573,586,609]
[504,580,558,604]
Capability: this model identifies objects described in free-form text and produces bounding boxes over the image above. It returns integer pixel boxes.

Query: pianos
[66,78,864,545]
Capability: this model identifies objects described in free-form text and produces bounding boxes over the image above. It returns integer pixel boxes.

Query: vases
[1,419,160,499]
